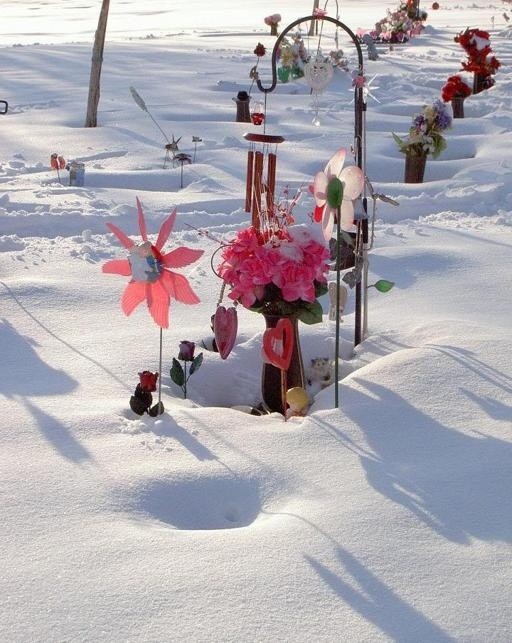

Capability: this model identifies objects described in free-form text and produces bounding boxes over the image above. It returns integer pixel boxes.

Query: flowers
[275,38,291,82]
[130,371,164,416]
[456,27,490,53]
[169,341,202,398]
[401,1,426,19]
[464,47,501,76]
[313,7,326,16]
[264,14,281,25]
[51,154,67,183]
[194,177,325,313]
[247,43,266,94]
[442,76,472,102]
[294,32,309,78]
[415,94,452,132]
[353,68,365,89]
[393,114,442,156]
[374,11,425,38]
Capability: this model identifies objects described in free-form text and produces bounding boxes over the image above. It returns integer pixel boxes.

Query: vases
[232,96,251,123]
[260,309,306,413]
[385,31,403,44]
[451,94,465,119]
[403,148,429,183]
[472,73,487,94]
[270,24,276,36]
